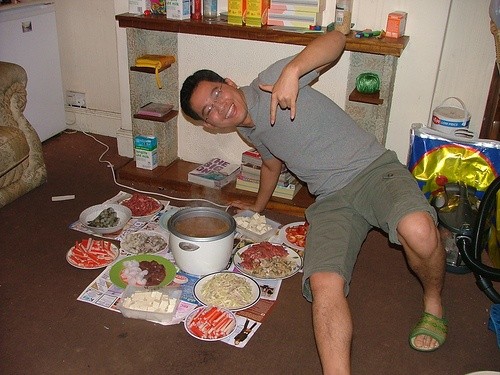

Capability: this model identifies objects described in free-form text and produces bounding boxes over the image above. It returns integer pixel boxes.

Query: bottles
[202,0,217,20]
[334,0,353,35]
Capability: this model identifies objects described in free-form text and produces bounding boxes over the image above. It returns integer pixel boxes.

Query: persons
[181,30,449,375]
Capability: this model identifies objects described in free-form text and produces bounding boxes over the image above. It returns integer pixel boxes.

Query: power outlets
[66,90,87,108]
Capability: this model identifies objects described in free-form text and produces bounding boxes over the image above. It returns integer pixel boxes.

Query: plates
[65,241,120,270]
[184,305,238,342]
[233,242,302,280]
[109,254,177,291]
[118,196,163,217]
[192,270,261,311]
[280,219,310,251]
[120,230,168,255]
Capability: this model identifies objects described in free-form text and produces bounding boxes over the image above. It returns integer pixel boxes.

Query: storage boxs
[387,12,406,38]
[228,0,326,29]
[166,0,190,20]
[135,134,159,171]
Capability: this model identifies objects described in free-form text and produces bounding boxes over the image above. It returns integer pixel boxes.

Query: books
[235,148,302,200]
[188,157,240,190]
[138,101,174,117]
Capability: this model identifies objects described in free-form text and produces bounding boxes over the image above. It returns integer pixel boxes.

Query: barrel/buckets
[432,96,471,138]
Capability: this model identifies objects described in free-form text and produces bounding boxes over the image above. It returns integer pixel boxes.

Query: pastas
[200,273,252,308]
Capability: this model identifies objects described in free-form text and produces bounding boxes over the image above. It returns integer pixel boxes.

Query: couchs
[0,62,49,210]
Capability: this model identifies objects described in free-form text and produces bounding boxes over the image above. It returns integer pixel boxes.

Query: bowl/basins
[79,203,132,233]
[116,285,181,322]
[231,209,282,242]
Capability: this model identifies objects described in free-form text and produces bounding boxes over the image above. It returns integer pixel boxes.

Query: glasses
[203,83,224,123]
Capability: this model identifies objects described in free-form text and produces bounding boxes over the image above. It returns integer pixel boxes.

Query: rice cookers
[167,206,238,275]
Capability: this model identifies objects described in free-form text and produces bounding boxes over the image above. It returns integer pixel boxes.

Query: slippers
[409,303,450,351]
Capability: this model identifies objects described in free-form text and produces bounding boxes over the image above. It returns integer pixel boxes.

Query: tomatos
[286,221,309,247]
